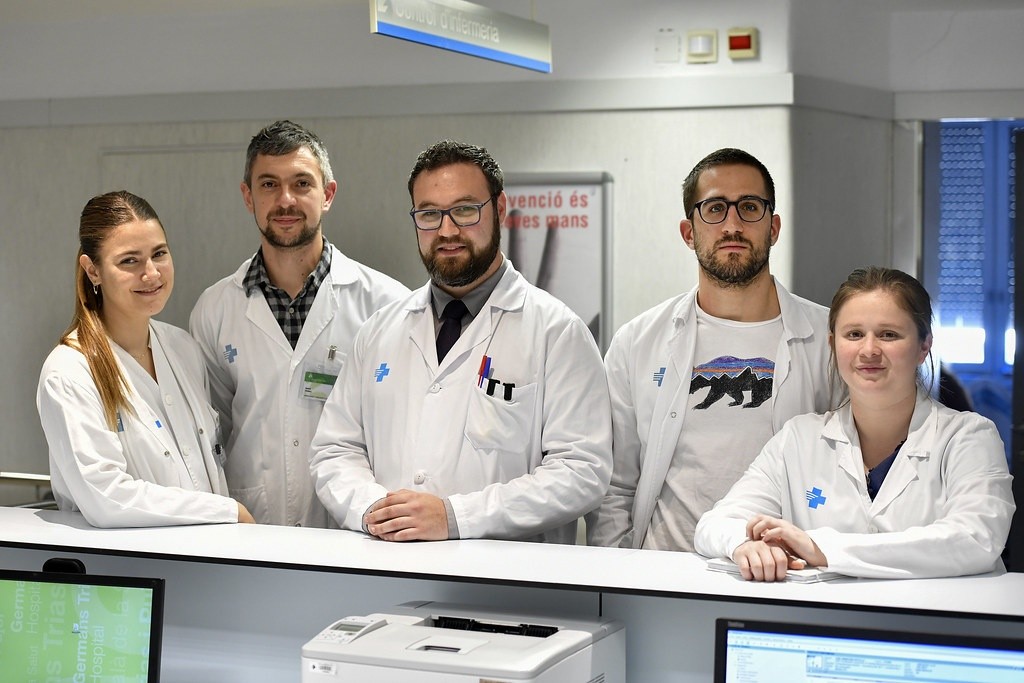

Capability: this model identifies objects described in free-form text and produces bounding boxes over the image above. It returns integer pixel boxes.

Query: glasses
[688,196,774,224]
[409,193,502,231]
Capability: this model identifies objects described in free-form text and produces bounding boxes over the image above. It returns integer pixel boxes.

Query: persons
[693,265,1017,583]
[308,138,613,543]
[189,119,413,530]
[36,189,258,527]
[584,147,852,552]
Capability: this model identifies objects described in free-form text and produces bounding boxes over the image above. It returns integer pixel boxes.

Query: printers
[300,598,627,683]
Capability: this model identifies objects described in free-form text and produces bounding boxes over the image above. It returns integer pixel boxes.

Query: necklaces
[131,347,149,358]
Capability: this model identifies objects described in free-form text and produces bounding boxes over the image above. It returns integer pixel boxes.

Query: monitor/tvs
[0,568,166,683]
[714,617,1024,683]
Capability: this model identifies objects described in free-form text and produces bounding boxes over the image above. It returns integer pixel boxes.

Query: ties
[436,300,469,366]
